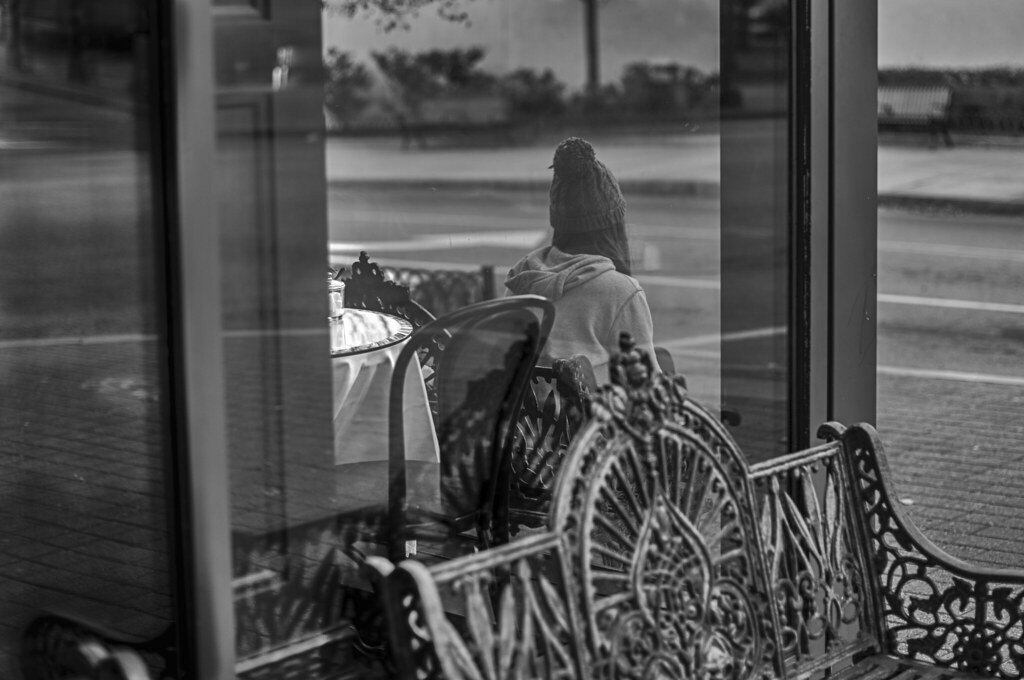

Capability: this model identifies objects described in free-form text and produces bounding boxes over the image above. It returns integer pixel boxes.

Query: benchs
[397,95,514,149]
[330,252,742,531]
[877,83,953,147]
[364,332,1024,680]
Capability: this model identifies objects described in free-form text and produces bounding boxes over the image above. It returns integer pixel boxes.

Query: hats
[548,137,626,233]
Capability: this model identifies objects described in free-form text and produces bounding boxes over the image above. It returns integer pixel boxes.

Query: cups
[327,272,345,322]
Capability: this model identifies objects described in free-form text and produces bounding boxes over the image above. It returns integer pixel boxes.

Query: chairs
[341,295,554,573]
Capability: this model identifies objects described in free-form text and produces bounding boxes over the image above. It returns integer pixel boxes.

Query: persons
[504,137,676,386]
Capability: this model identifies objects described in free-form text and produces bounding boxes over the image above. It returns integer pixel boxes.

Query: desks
[329,307,443,522]
